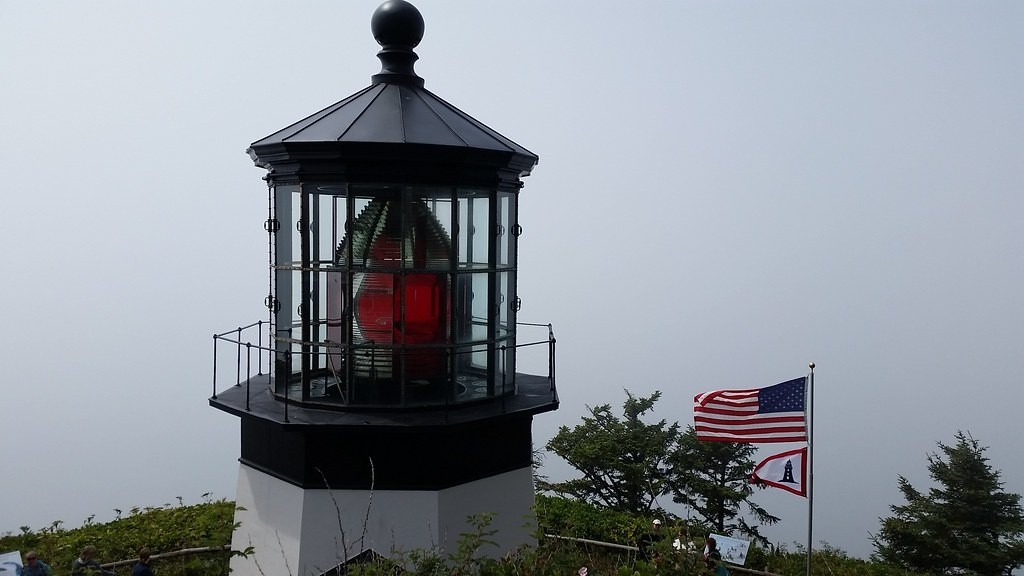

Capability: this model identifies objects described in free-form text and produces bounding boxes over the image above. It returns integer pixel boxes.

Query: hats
[653,519,661,525]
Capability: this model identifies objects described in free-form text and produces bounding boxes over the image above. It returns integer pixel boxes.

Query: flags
[694,374,810,442]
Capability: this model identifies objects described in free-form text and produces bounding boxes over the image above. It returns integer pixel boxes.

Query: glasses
[27,556,36,561]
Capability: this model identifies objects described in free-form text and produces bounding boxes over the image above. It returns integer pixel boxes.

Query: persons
[646,517,724,564]
